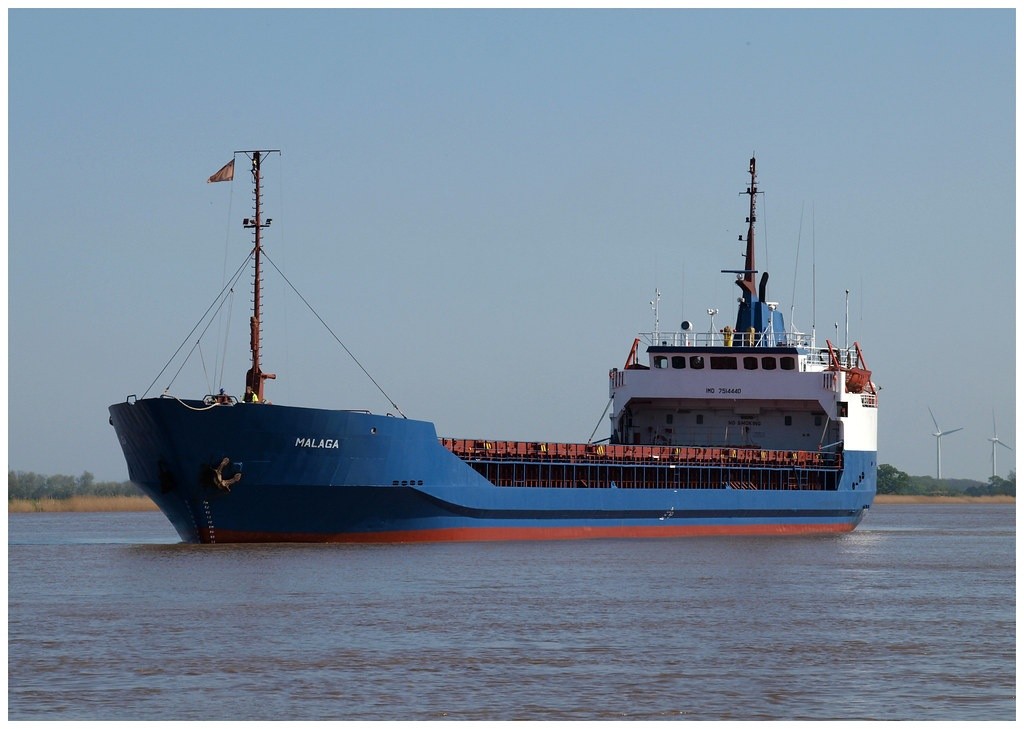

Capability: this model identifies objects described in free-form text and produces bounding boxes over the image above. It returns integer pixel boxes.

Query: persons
[212,388,232,402]
[240,386,258,405]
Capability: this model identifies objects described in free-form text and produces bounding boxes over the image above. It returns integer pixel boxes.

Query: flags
[206,158,235,183]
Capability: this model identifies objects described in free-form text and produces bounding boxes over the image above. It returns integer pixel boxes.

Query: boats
[107,147,876,544]
